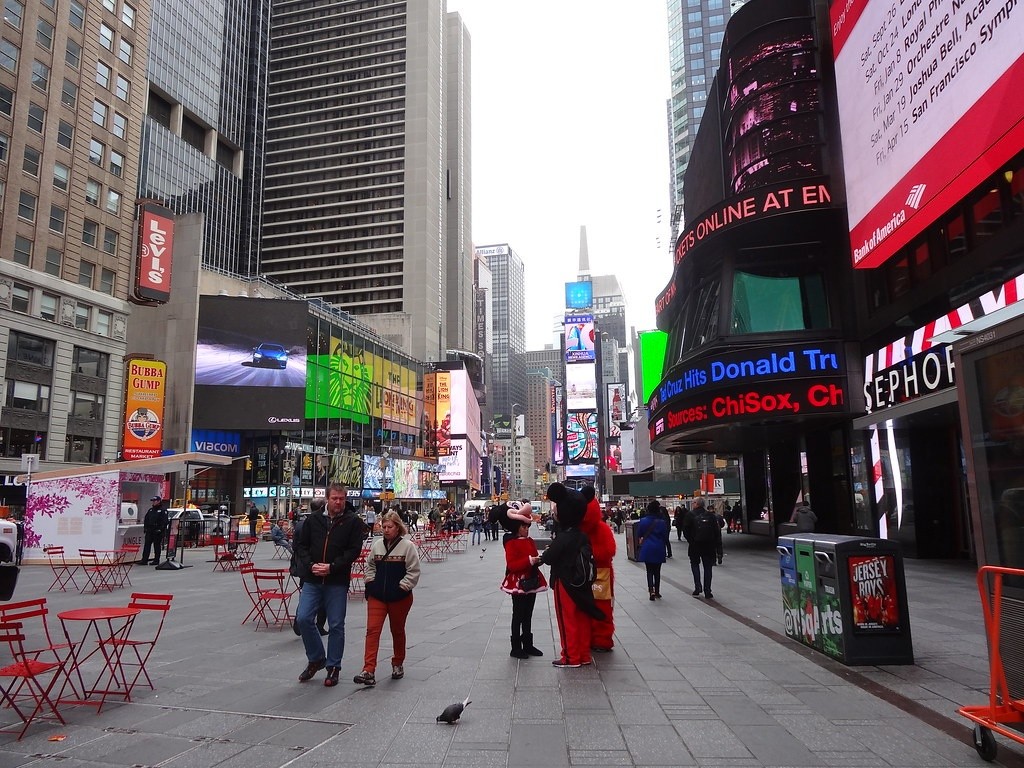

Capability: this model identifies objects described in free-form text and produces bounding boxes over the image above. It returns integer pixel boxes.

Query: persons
[427,502,499,545]
[607,446,621,472]
[568,324,587,351]
[488,499,544,659]
[635,501,669,602]
[792,500,817,532]
[291,500,328,637]
[382,505,419,532]
[138,495,169,565]
[271,518,294,555]
[352,509,421,685]
[295,486,363,687]
[247,498,259,537]
[683,496,720,600]
[289,505,303,522]
[540,494,742,535]
[366,506,377,537]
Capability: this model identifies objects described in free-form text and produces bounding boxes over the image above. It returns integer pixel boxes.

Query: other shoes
[655,594,662,598]
[650,592,655,600]
[293,615,302,636]
[318,628,329,636]
[552,658,581,667]
[705,592,713,598]
[136,558,148,564]
[149,559,159,565]
[692,585,702,595]
[510,636,528,658]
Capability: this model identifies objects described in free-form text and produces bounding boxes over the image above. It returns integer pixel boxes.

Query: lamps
[273,283,287,288]
[253,274,267,279]
[297,293,308,298]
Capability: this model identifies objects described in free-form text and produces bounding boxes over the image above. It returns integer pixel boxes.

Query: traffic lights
[303,456,312,469]
[246,459,252,471]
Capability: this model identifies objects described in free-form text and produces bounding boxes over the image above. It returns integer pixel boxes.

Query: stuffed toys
[537,482,616,670]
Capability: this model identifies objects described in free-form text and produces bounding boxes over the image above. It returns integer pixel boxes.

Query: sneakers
[353,670,376,685]
[299,657,327,680]
[392,665,403,679]
[324,667,340,686]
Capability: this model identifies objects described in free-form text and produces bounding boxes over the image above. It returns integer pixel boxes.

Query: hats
[150,496,160,501]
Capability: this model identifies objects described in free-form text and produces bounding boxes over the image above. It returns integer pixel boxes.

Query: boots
[522,633,543,656]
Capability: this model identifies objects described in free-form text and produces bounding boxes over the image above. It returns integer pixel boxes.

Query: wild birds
[480,555,483,559]
[482,548,487,552]
[435,696,472,725]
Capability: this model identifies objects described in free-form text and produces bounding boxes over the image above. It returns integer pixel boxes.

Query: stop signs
[262,522,272,531]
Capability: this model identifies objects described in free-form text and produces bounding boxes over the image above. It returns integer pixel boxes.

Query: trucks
[0,517,24,601]
[530,501,542,515]
[463,499,492,512]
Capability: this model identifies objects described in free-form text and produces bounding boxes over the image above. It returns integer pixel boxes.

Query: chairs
[102,544,143,589]
[79,549,112,594]
[206,534,315,632]
[43,546,78,592]
[0,622,66,741]
[347,546,373,597]
[88,592,173,702]
[0,597,82,713]
[412,524,471,563]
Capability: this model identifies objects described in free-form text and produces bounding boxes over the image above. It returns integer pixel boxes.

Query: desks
[226,539,257,572]
[447,532,464,552]
[57,607,141,713]
[88,549,127,592]
[254,566,294,623]
[419,535,443,560]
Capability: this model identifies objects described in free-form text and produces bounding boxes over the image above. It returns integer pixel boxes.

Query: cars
[252,343,290,369]
[200,512,266,536]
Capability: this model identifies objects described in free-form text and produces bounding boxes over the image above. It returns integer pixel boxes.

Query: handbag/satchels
[522,578,539,591]
[486,523,492,530]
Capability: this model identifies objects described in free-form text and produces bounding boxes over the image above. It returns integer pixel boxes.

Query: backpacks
[472,515,481,526]
[690,510,715,541]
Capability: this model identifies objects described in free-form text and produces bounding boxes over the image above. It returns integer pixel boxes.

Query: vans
[166,507,204,532]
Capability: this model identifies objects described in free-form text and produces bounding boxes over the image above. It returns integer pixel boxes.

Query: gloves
[672,520,678,526]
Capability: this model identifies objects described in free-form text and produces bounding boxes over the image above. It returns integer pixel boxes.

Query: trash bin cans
[625,520,645,561]
[776,534,914,666]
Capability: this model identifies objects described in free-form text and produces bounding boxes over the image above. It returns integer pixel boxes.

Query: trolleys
[957,564,1024,762]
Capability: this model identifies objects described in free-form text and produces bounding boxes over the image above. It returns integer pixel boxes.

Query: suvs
[464,511,487,532]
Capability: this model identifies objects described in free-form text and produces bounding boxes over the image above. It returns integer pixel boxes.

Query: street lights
[289,444,303,526]
[511,403,520,501]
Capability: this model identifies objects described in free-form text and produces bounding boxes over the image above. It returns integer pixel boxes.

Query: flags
[35,435,42,442]
